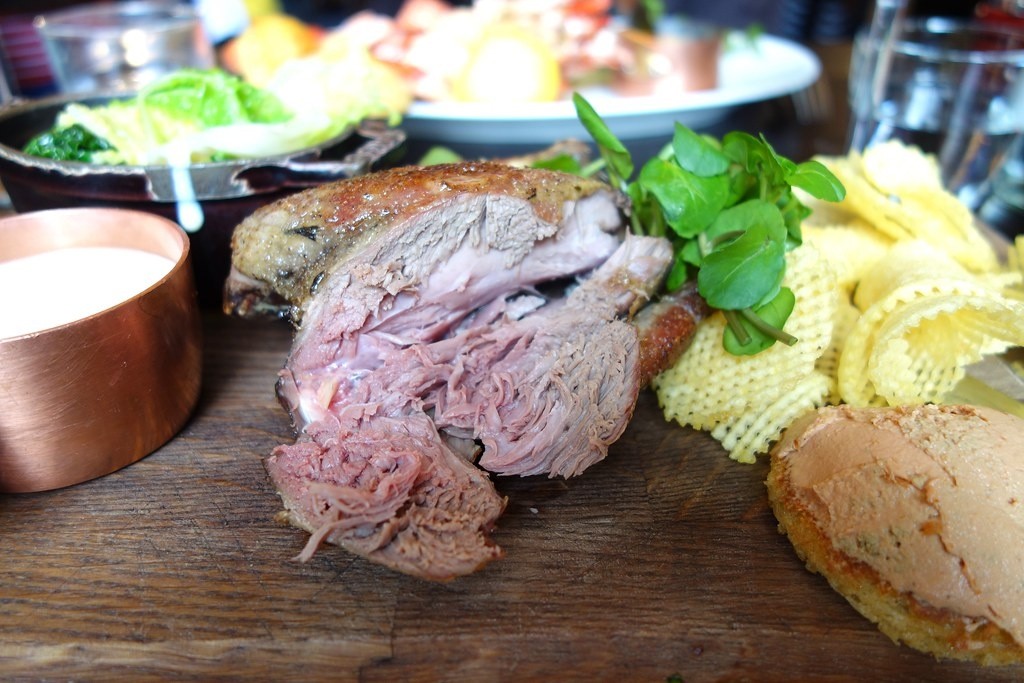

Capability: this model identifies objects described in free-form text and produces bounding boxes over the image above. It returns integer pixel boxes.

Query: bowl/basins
[0,204,203,494]
[32,0,215,102]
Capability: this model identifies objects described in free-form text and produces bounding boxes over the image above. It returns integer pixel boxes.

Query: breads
[767,403,1024,667]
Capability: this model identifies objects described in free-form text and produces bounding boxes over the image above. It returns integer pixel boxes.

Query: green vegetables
[536,90,845,354]
[26,67,319,169]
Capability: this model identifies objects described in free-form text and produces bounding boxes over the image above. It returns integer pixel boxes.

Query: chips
[649,139,1024,463]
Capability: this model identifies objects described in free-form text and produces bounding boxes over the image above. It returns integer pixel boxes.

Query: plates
[389,25,823,143]
[1,84,407,203]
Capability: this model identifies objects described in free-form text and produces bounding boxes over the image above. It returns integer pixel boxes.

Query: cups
[843,0,1024,242]
